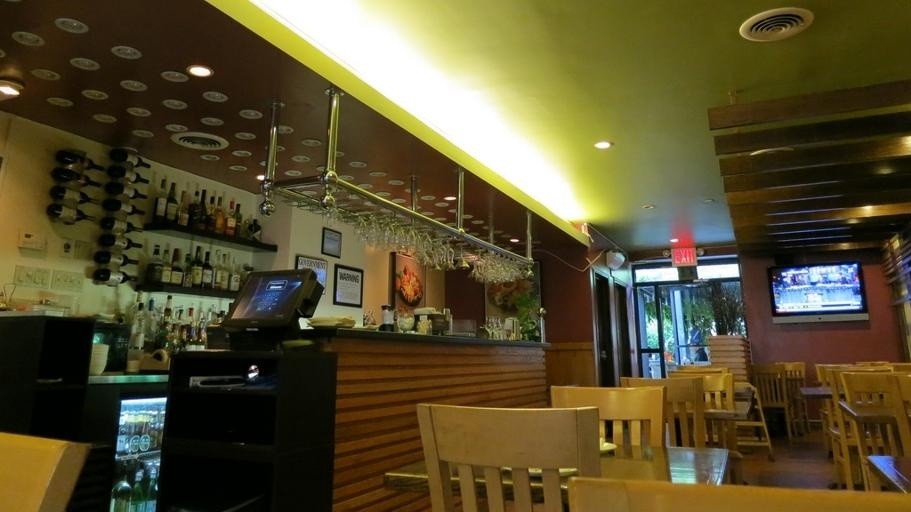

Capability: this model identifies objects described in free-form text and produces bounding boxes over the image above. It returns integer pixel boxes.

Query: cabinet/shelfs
[134,223,276,297]
[1,314,97,440]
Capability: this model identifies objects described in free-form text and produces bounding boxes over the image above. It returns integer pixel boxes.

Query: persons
[693,341,710,362]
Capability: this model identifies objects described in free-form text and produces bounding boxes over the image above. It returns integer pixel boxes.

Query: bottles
[483,313,506,340]
[48,149,107,225]
[146,175,263,243]
[92,148,152,284]
[109,409,167,512]
[126,290,226,374]
[144,240,254,292]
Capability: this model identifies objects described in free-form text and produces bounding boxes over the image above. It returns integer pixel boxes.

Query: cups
[90,342,110,376]
[398,313,416,331]
[417,320,433,335]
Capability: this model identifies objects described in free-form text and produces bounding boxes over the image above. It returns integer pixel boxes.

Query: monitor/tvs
[766,260,869,324]
[219,269,324,335]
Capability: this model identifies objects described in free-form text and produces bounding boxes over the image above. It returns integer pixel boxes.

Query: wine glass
[354,213,535,285]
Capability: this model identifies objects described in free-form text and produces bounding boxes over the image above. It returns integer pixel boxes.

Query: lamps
[604,248,628,271]
